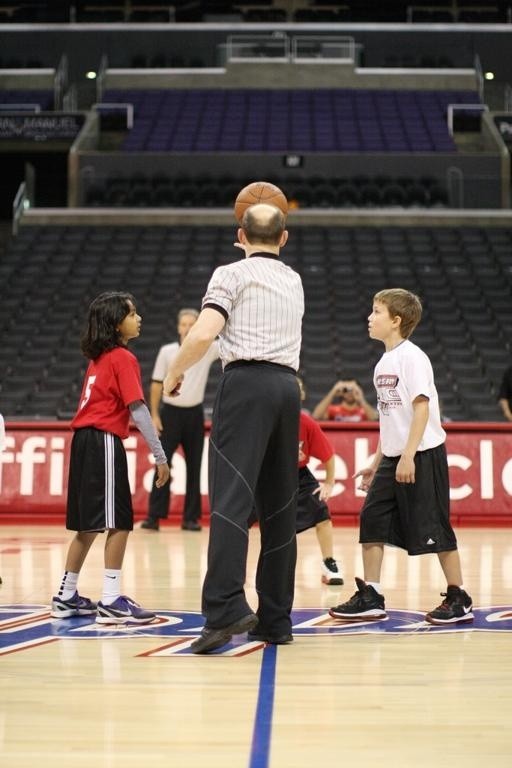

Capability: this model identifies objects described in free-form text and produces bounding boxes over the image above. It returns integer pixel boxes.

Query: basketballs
[235,182,288,228]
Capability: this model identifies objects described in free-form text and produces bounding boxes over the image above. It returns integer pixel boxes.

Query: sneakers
[425,585,475,626]
[248,628,293,643]
[140,521,159,530]
[182,520,201,530]
[191,614,259,654]
[328,578,387,618]
[50,591,96,618]
[321,557,343,584]
[95,595,155,623]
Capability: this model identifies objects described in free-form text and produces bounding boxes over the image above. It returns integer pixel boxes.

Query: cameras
[340,385,352,392]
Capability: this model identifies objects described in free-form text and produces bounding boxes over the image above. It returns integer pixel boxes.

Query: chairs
[0,88,512,420]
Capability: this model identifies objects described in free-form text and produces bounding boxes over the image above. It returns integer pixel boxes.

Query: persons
[327,287,477,623]
[162,201,305,652]
[141,307,220,531]
[498,363,512,422]
[50,291,170,624]
[247,377,346,585]
[310,379,381,423]
